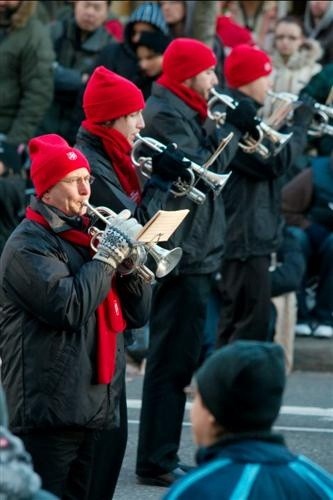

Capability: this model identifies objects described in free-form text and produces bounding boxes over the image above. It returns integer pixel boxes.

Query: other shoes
[313,324,333,339]
[295,324,312,336]
[137,464,197,487]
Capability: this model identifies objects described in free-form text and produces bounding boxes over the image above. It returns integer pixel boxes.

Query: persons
[0,425,61,500]
[0,134,152,500]
[0,0,332,487]
[161,343,333,500]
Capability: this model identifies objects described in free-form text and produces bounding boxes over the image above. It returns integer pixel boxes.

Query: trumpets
[130,132,233,206]
[81,198,182,284]
[206,87,294,159]
[265,89,333,136]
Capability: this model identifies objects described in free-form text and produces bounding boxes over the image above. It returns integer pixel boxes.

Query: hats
[83,66,146,124]
[135,27,169,54]
[214,16,256,49]
[224,44,273,89]
[195,341,288,431]
[162,39,217,83]
[28,134,91,200]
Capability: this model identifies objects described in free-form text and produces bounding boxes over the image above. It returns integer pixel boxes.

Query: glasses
[274,34,298,41]
[58,175,95,186]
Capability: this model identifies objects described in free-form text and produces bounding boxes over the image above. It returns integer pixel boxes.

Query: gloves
[292,93,321,128]
[93,209,143,268]
[226,98,261,136]
[151,142,192,183]
